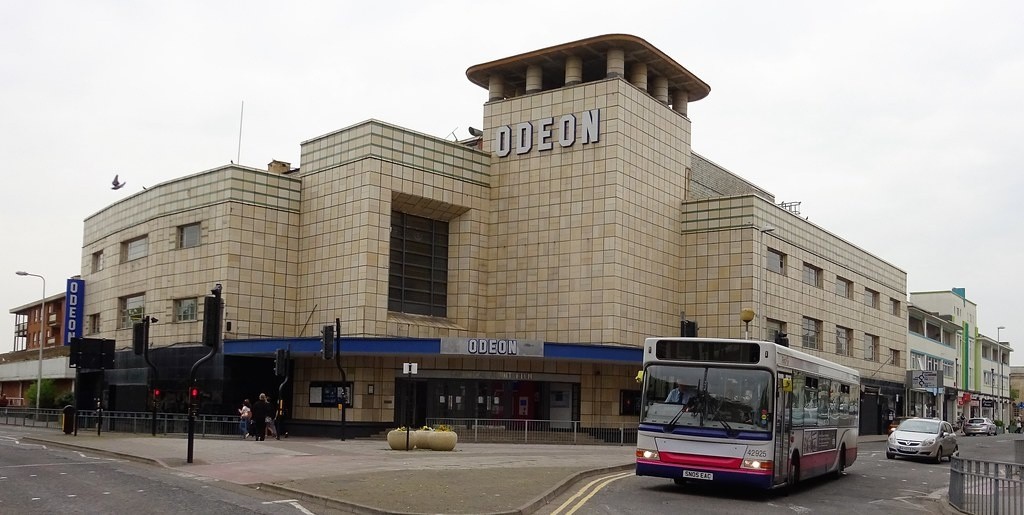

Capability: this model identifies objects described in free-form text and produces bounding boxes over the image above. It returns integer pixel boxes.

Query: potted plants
[428,425,458,451]
[416,426,435,449]
[387,427,417,450]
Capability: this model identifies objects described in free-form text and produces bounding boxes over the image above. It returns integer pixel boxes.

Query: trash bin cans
[62,405,75,434]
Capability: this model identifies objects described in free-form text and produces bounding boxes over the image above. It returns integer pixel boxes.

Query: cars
[888,416,919,437]
[963,417,998,436]
[886,418,960,465]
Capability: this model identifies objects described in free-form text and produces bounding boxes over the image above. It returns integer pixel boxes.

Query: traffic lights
[963,394,971,400]
[153,389,161,408]
[319,325,333,360]
[189,386,198,415]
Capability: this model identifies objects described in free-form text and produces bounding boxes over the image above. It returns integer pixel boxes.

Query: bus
[635,337,862,497]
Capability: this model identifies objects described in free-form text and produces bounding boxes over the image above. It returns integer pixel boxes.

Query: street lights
[740,307,755,340]
[759,226,775,337]
[998,326,1006,428]
[16,270,46,421]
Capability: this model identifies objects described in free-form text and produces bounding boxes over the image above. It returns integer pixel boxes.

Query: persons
[238,393,289,442]
[960,413,966,433]
[665,376,701,413]
[0,394,8,416]
[1013,414,1023,433]
